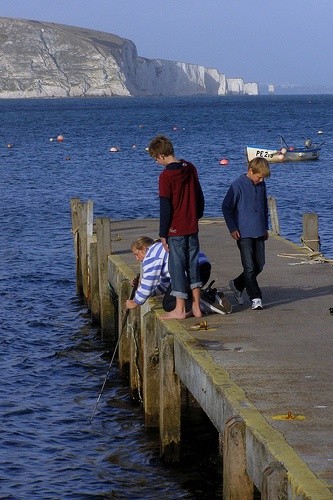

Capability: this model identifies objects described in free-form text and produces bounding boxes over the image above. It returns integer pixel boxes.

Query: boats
[245,136,326,164]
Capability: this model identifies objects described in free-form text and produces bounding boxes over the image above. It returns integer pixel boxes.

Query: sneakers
[229,280,244,304]
[251,298,263,309]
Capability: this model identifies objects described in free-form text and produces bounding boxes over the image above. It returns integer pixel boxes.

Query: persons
[222,157,272,310]
[149,136,206,320]
[125,236,233,315]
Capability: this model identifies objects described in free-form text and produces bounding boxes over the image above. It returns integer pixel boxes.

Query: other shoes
[215,292,232,314]
[200,297,226,315]
